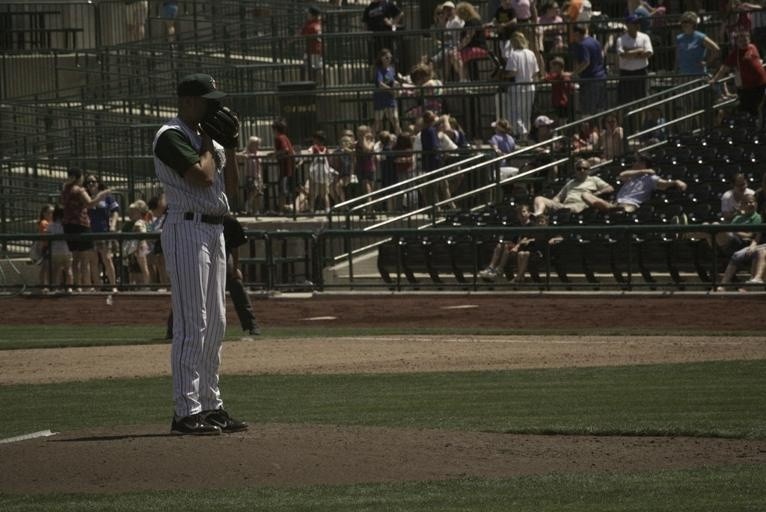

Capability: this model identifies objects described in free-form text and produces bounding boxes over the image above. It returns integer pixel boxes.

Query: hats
[625,14,641,25]
[491,120,504,131]
[175,72,228,102]
[534,115,554,129]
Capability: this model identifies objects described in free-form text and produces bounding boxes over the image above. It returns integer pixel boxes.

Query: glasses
[576,166,588,172]
[87,181,97,184]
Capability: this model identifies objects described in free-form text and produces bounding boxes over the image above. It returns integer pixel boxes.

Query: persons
[165,216,260,337]
[152,73,249,436]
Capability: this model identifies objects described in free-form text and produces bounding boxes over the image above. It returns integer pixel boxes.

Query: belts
[185,212,225,225]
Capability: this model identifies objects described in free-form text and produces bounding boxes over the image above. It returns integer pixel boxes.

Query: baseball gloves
[202,104,239,147]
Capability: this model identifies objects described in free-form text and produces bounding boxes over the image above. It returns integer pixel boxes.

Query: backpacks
[311,147,331,185]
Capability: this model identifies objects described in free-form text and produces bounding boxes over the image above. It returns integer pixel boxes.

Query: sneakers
[485,270,503,278]
[40,287,118,295]
[249,327,260,335]
[479,268,491,276]
[203,409,250,432]
[170,412,222,435]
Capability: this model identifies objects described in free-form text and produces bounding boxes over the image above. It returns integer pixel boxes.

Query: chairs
[376,101,766,274]
